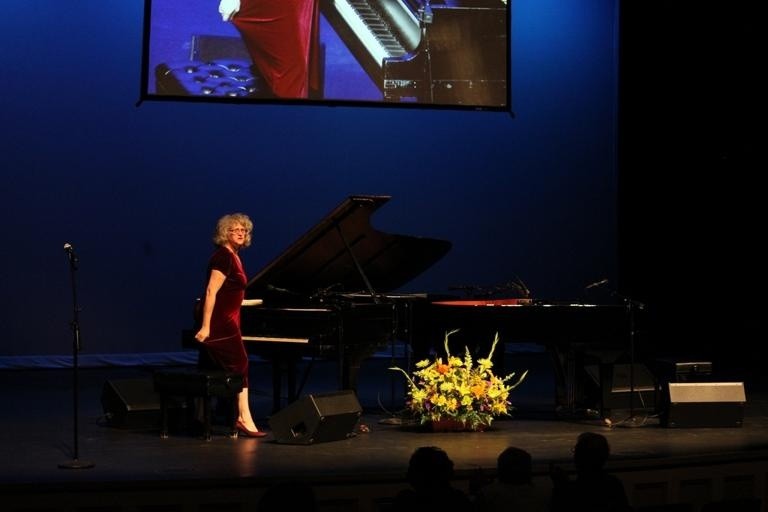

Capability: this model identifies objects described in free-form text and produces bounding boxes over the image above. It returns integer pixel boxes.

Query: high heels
[235,420,268,437]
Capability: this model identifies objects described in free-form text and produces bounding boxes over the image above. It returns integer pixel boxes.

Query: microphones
[585,278,609,291]
[64,242,80,261]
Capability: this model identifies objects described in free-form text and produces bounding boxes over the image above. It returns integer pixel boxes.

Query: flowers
[387,325,529,433]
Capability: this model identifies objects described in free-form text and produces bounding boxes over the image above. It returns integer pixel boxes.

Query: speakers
[100,375,162,429]
[606,364,655,412]
[659,381,746,427]
[270,392,364,445]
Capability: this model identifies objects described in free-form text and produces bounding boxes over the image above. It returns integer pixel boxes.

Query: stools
[153,372,242,441]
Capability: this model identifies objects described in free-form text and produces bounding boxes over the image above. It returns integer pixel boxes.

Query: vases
[430,417,484,434]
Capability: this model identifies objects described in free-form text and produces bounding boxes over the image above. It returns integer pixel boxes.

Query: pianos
[319,0,507,107]
[183,194,452,410]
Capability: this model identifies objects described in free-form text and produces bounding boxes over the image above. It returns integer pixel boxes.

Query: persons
[373,446,476,511]
[479,446,546,511]
[216,0,321,99]
[550,431,631,511]
[191,211,269,438]
